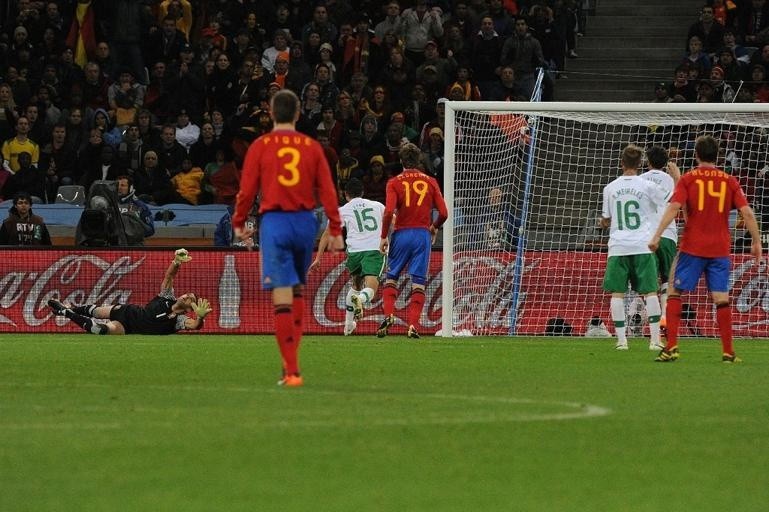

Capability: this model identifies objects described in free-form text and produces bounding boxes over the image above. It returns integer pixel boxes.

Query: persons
[648,135,763,364]
[48,248,213,336]
[309,177,396,336]
[637,146,677,331]
[0,1,585,252]
[231,89,345,387]
[637,0,769,256]
[376,142,448,339]
[598,144,681,352]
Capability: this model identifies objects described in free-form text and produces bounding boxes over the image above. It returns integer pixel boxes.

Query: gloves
[191,298,212,321]
[173,248,193,267]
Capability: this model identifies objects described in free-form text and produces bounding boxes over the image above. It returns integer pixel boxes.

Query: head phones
[129,184,134,193]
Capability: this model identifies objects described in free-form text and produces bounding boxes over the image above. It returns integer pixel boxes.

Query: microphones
[121,189,135,202]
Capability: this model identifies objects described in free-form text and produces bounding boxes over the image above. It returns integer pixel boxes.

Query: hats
[429,98,449,138]
[370,155,384,165]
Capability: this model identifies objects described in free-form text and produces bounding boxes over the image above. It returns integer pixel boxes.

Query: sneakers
[649,342,678,361]
[277,375,303,386]
[408,330,420,338]
[48,299,74,315]
[616,342,628,350]
[351,294,363,319]
[723,353,742,363]
[378,316,394,337]
